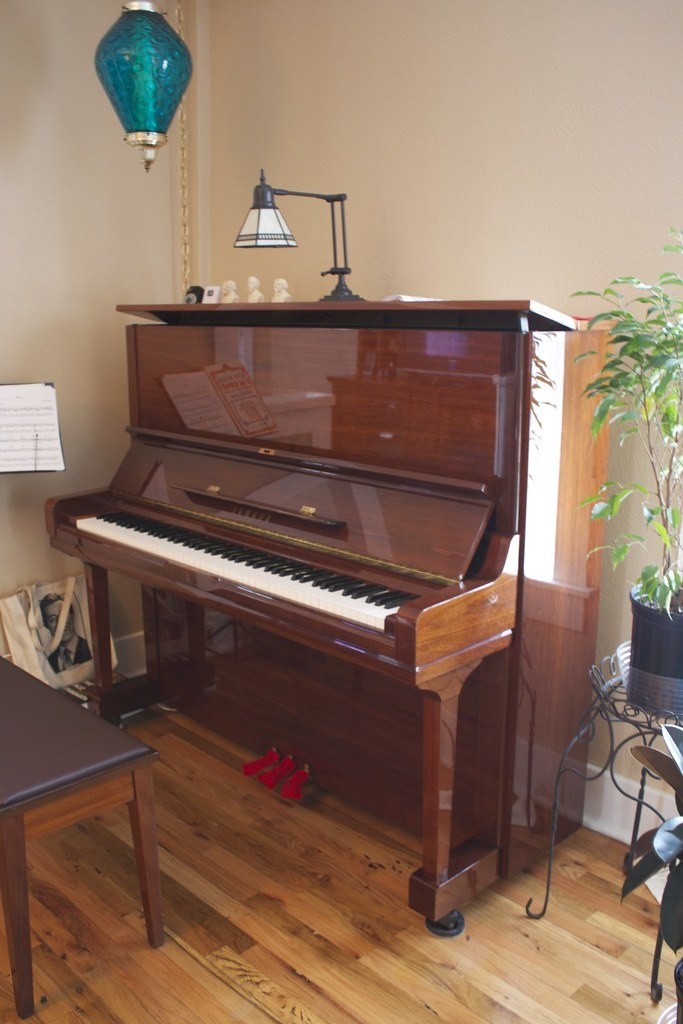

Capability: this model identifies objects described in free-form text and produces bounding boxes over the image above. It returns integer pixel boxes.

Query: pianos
[41,298,624,939]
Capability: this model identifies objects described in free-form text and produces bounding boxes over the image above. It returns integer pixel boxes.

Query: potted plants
[619,724,683,1024]
[568,224,683,720]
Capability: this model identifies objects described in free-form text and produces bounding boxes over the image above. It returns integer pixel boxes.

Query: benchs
[0,655,166,1019]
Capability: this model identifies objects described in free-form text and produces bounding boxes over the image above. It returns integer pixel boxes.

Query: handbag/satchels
[0,572,119,690]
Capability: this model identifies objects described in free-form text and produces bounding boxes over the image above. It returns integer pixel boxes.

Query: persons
[38,590,92,673]
[247,275,265,303]
[219,279,239,302]
[271,277,290,302]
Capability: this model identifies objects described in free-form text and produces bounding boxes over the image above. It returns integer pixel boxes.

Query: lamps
[95,1,193,173]
[232,168,365,302]
